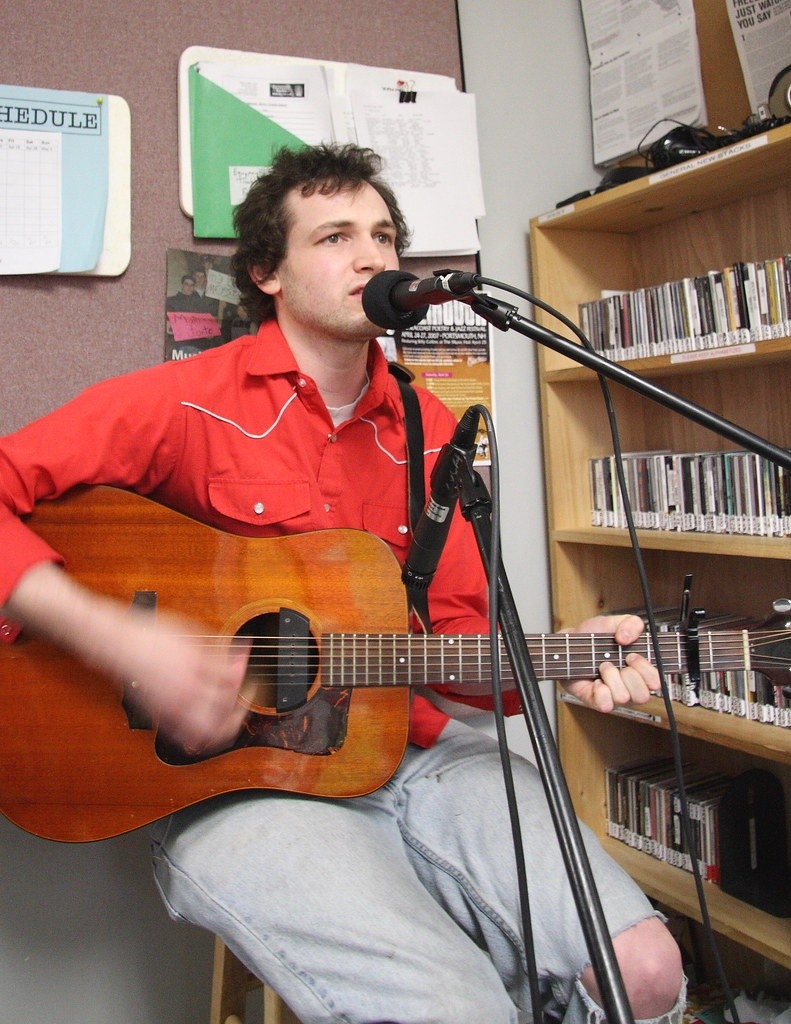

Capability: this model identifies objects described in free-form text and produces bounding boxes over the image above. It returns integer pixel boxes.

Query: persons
[1,144,687,1024]
[167,257,258,353]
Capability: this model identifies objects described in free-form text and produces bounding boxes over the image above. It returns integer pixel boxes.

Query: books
[587,448,791,538]
[601,603,791,730]
[578,252,791,362]
[603,749,735,884]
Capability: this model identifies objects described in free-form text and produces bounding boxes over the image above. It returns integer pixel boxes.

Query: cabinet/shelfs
[529,121,791,1024]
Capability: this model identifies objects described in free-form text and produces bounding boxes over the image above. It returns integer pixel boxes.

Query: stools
[210,932,305,1024]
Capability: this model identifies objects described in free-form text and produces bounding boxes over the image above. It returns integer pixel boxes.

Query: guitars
[2,487,791,842]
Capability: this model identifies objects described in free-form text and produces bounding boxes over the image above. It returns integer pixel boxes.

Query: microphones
[362,269,480,329]
[401,405,481,591]
